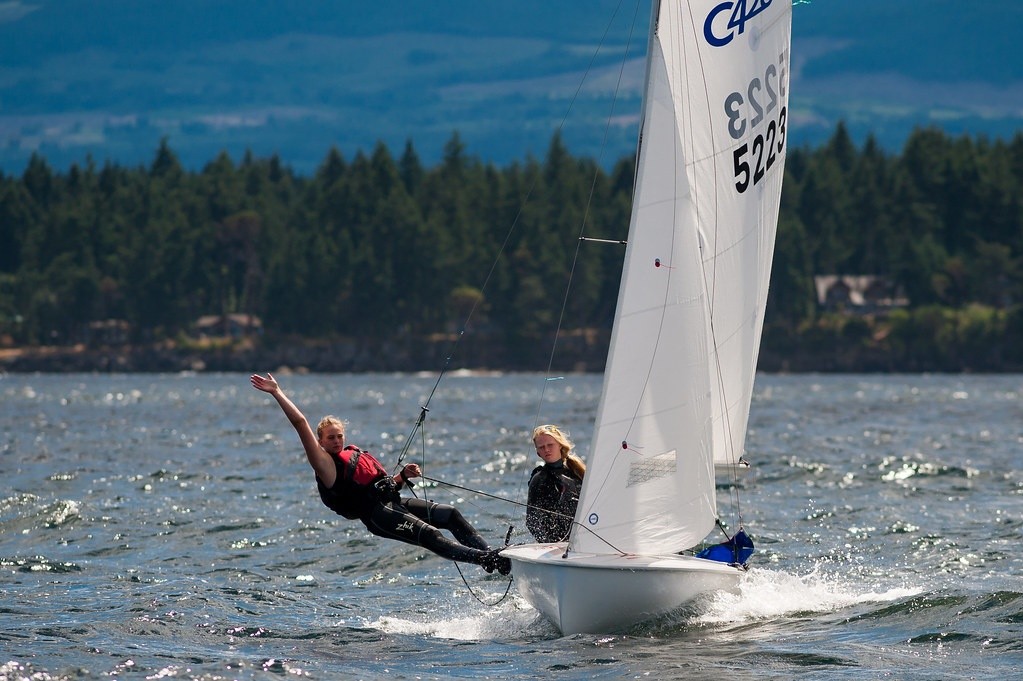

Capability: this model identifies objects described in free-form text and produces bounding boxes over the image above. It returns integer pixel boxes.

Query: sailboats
[395,0,797,638]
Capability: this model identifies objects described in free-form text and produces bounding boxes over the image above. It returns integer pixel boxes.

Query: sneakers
[478,542,526,576]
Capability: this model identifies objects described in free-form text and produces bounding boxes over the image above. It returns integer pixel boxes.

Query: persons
[251,373,525,575]
[526,424,586,543]
